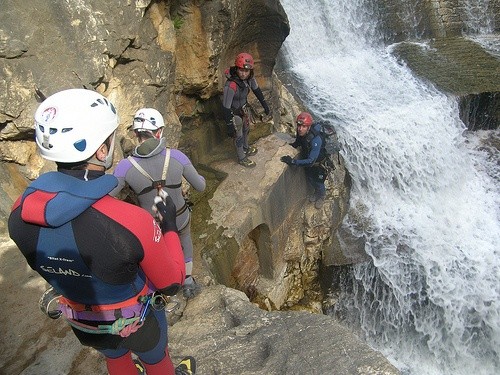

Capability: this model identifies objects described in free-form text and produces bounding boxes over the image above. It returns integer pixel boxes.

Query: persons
[220,53,269,167]
[108,107,207,298]
[7,89,196,375]
[280,112,332,208]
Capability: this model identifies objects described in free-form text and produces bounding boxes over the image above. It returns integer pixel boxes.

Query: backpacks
[306,120,341,154]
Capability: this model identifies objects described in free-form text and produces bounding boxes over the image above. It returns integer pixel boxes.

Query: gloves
[152,189,179,233]
[280,154,293,166]
[289,140,300,148]
[262,100,270,114]
[225,120,236,136]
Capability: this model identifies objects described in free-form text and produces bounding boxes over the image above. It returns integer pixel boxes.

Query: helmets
[35,89,119,163]
[133,108,165,130]
[295,112,313,126]
[235,53,255,69]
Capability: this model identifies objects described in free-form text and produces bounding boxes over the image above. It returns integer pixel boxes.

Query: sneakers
[240,158,257,168]
[245,147,258,156]
[133,359,144,375]
[175,355,196,375]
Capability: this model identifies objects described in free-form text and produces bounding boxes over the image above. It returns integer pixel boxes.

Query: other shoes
[309,190,324,209]
[183,284,202,297]
